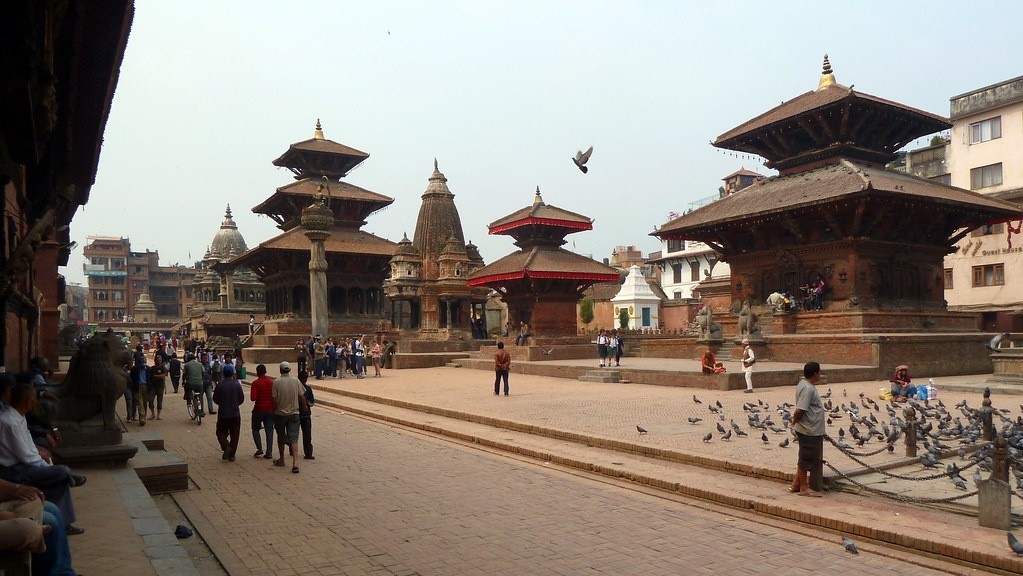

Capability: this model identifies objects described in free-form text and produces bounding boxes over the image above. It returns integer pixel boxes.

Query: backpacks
[351,341,362,353]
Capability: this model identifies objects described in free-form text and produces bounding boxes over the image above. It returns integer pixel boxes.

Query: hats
[393,341,397,345]
[742,339,749,344]
[223,366,234,376]
[383,340,388,343]
[279,361,290,372]
[168,341,172,345]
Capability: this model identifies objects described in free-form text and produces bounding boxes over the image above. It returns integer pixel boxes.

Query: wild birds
[636,425,647,434]
[702,432,713,443]
[688,417,702,424]
[842,536,859,555]
[693,395,702,404]
[708,388,1023,491]
[1007,527,1023,556]
[571,146,593,174]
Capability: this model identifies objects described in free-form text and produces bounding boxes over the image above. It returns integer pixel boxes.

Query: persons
[701,349,725,375]
[294,334,398,380]
[493,341,511,397]
[784,273,825,310]
[212,361,315,475]
[248,314,255,335]
[107,328,247,354]
[515,321,530,346]
[788,360,826,498]
[122,313,133,322]
[740,338,756,393]
[122,352,246,426]
[596,328,625,367]
[0,357,86,576]
[478,321,487,339]
[890,364,917,403]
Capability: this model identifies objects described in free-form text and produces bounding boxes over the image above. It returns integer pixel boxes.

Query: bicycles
[181,382,202,425]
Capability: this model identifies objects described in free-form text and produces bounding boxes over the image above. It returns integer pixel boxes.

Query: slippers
[292,467,299,473]
[274,459,285,466]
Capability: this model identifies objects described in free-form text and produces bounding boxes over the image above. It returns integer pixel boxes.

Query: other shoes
[157,416,163,420]
[317,370,381,380]
[222,447,231,460]
[264,454,273,459]
[65,523,85,535]
[305,455,315,460]
[744,389,752,393]
[598,362,621,367]
[71,474,86,488]
[228,457,234,461]
[140,421,146,426]
[133,417,138,421]
[208,410,217,415]
[126,419,132,423]
[254,450,263,458]
[148,414,155,421]
